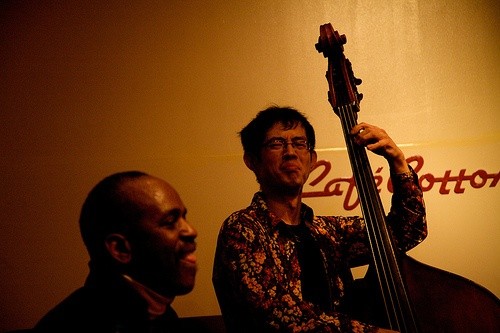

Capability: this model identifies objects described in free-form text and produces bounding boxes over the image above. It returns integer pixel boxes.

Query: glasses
[262,140,314,154]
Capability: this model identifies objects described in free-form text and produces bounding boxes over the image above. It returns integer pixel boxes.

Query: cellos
[315,23,499,333]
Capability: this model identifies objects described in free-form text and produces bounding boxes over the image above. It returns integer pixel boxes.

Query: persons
[28,171,198,332]
[212,106,428,333]
